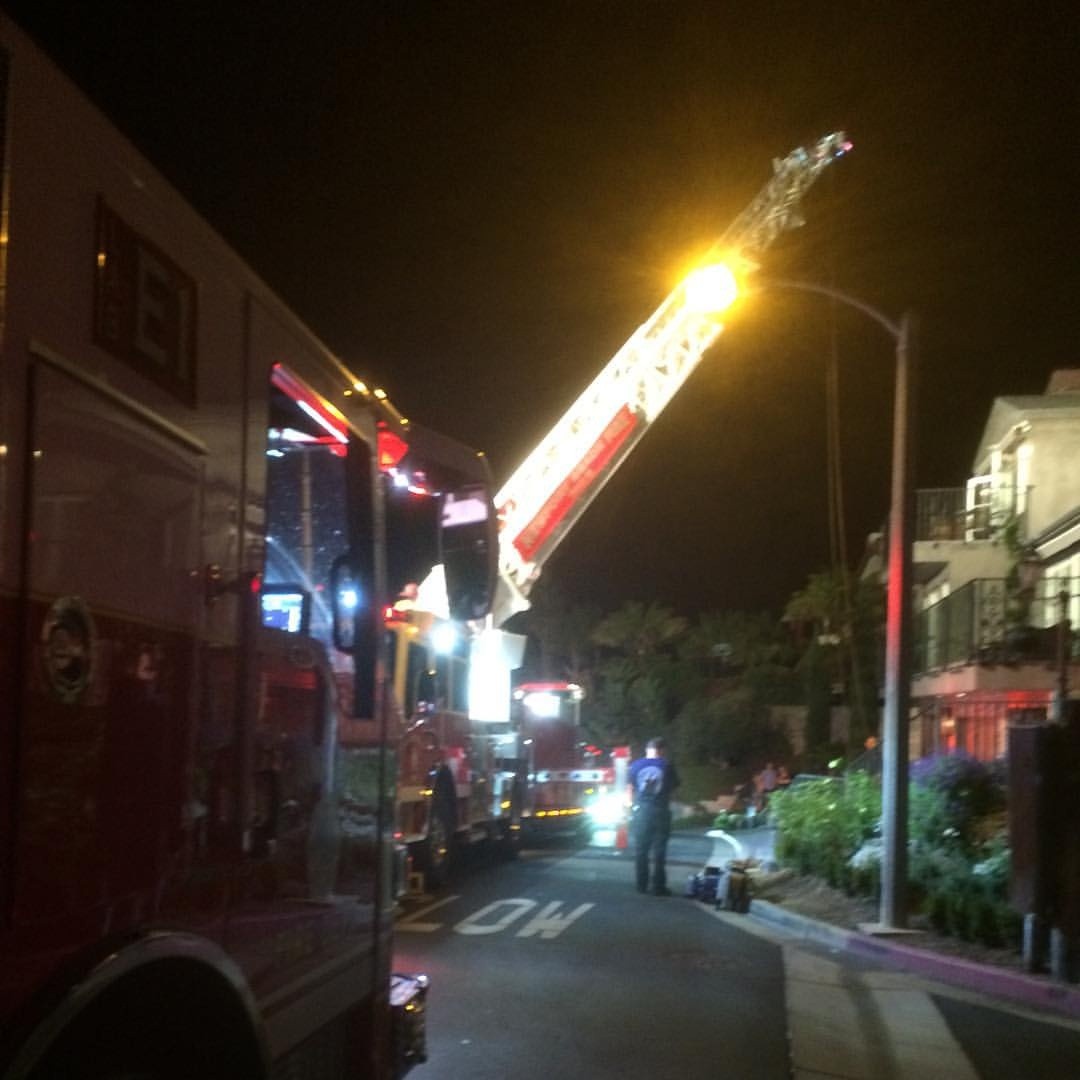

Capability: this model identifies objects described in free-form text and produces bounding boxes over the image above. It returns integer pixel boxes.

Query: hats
[645,740,663,750]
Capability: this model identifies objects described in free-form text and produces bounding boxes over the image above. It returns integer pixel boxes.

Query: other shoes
[655,886,671,897]
[637,886,647,894]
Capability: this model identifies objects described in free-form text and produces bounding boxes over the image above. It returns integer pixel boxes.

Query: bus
[0,15,501,1080]
[382,605,591,890]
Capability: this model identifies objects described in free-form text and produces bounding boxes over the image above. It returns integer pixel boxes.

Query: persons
[626,736,679,896]
[758,760,791,793]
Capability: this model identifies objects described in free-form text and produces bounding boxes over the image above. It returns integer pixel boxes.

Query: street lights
[683,261,915,928]
